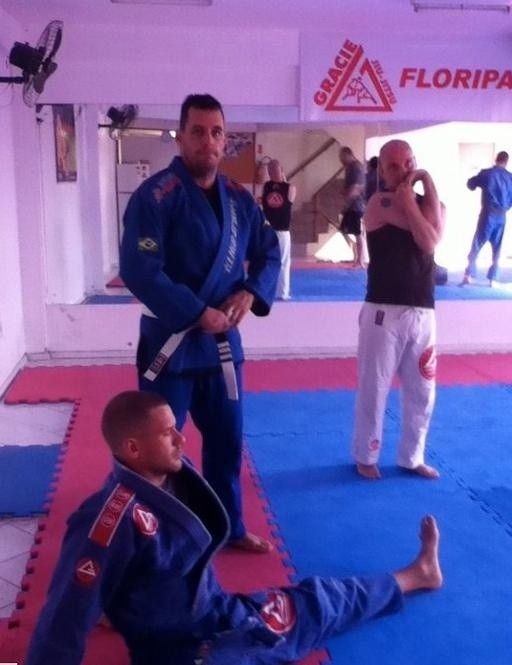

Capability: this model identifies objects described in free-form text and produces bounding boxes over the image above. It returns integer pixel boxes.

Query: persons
[23,390,443,665]
[353,139,443,480]
[339,146,368,270]
[119,93,281,553]
[365,156,385,200]
[261,160,297,300]
[456,152,512,287]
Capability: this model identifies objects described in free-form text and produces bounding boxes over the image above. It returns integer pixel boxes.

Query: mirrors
[35,98,509,306]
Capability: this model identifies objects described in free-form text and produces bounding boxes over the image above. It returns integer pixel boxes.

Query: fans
[1,16,63,111]
[106,103,140,139]
